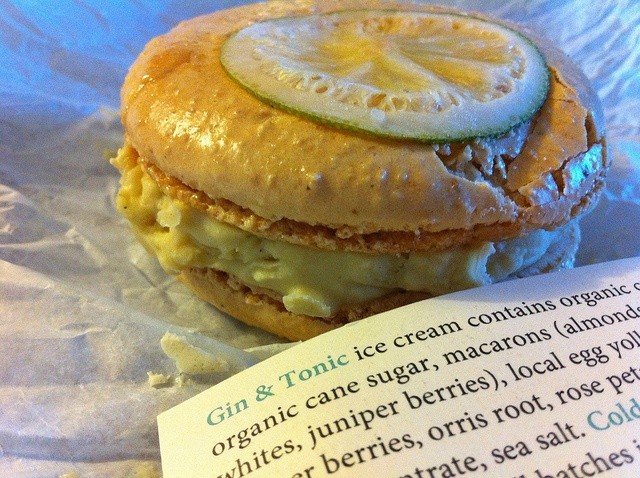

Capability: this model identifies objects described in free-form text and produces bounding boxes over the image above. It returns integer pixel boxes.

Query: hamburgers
[109,0,614,341]
